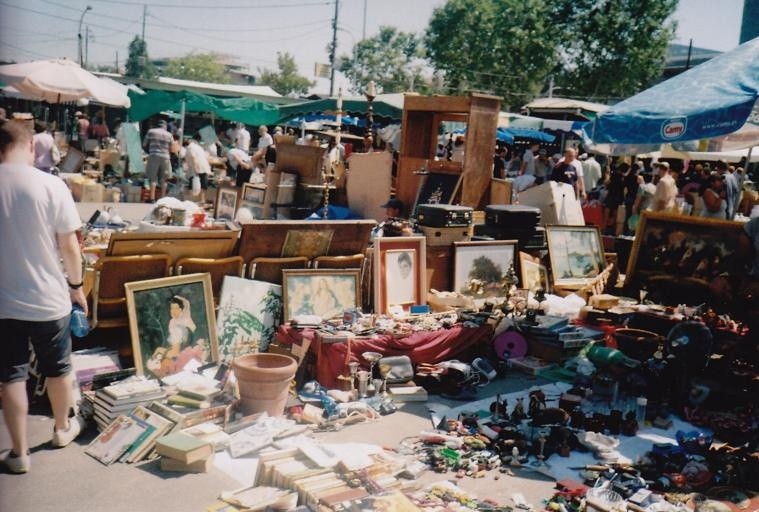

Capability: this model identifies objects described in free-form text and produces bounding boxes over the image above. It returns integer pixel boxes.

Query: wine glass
[347,351,391,400]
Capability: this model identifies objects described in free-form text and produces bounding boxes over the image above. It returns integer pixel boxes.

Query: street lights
[77,4,91,68]
[331,25,359,95]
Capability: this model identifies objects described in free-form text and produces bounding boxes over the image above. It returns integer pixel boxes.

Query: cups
[192,212,205,227]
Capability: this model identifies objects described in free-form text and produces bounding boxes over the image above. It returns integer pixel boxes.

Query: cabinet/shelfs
[396,90,505,210]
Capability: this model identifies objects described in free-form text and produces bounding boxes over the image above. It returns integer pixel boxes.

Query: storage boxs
[57,149,142,206]
[513,179,588,227]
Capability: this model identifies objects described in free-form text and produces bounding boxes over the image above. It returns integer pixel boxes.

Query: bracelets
[67,280,83,289]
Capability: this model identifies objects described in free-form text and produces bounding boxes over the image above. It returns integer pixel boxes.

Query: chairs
[83,250,172,333]
[269,186,326,221]
[174,253,246,318]
[313,252,368,289]
[248,256,312,286]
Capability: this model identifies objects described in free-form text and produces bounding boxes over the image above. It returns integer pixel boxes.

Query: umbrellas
[0,57,131,109]
[583,36,759,157]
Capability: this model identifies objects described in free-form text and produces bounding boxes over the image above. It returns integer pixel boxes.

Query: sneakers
[53,415,86,447]
[0,448,32,474]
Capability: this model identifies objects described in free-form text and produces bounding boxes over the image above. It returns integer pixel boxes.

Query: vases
[609,326,662,365]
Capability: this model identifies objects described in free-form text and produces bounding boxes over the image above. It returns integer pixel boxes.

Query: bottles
[172,208,186,226]
[70,304,90,338]
[635,395,648,427]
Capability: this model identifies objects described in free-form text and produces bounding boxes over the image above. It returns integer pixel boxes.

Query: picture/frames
[372,234,429,317]
[281,266,363,326]
[543,222,610,291]
[123,272,222,379]
[624,207,757,322]
[517,248,550,297]
[447,238,522,294]
[212,180,271,220]
[414,166,464,207]
[428,113,469,162]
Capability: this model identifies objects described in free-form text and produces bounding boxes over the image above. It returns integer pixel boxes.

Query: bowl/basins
[611,326,660,361]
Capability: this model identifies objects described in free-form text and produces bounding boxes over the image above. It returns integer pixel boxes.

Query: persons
[381,199,405,217]
[1,117,89,472]
[436,134,758,252]
[33,111,400,204]
